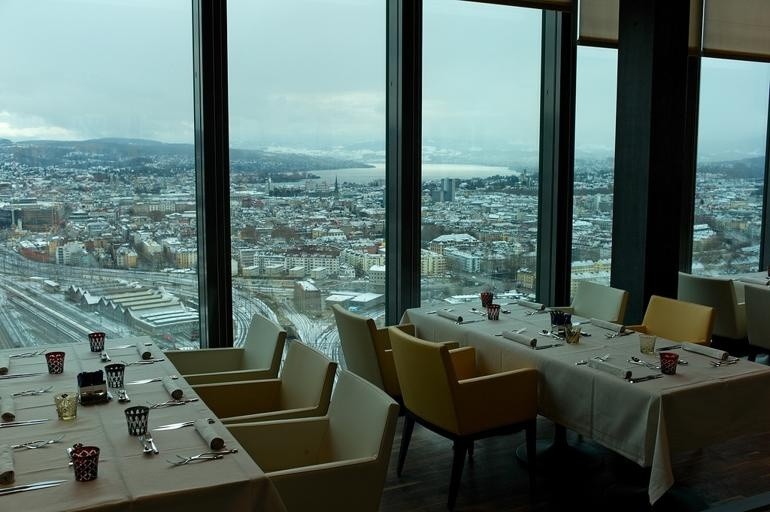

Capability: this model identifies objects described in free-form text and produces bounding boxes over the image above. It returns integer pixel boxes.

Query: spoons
[629,356,659,370]
[122,358,154,365]
[137,434,152,454]
[542,329,564,342]
[470,307,487,317]
[115,389,126,404]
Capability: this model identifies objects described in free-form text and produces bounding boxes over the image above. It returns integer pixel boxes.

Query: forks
[146,398,201,411]
[495,327,527,336]
[709,358,739,366]
[525,308,553,315]
[426,306,454,317]
[143,430,159,455]
[575,354,610,365]
[605,330,635,338]
[165,455,224,465]
[11,386,52,397]
[7,433,65,449]
[9,349,47,358]
[176,448,238,458]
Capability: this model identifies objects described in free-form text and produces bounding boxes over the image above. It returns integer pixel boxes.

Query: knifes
[109,342,154,350]
[0,419,50,428]
[154,418,214,431]
[656,342,688,352]
[0,371,42,378]
[128,375,180,385]
[630,372,661,383]
[0,479,68,494]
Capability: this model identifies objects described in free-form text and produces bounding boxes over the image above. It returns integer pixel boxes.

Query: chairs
[332,302,460,415]
[388,322,540,511]
[226,371,399,511]
[164,313,287,385]
[743,282,770,363]
[624,295,715,345]
[549,282,631,322]
[189,341,337,424]
[677,272,746,340]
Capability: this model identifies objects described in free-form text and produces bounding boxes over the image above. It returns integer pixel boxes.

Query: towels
[437,310,463,322]
[0,446,14,483]
[136,341,151,359]
[589,359,632,378]
[740,277,768,284]
[682,342,727,359]
[1,394,15,422]
[162,376,183,399]
[592,319,624,332]
[0,353,8,374]
[195,420,224,450]
[518,301,544,310]
[503,331,537,346]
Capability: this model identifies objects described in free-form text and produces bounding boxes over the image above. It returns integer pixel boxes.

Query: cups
[487,303,499,320]
[72,446,100,481]
[55,391,79,421]
[564,325,581,344]
[660,352,678,375]
[124,405,149,436]
[638,334,656,354]
[104,363,126,387]
[481,292,494,307]
[87,332,105,351]
[46,351,65,373]
